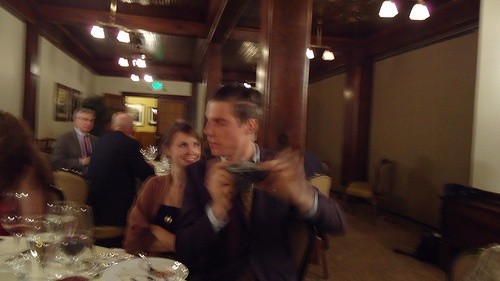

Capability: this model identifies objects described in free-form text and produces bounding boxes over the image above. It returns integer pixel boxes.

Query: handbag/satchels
[414,230,450,270]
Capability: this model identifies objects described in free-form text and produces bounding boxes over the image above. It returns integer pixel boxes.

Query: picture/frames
[53,82,71,122]
[125,102,145,127]
[70,88,83,122]
[149,107,158,126]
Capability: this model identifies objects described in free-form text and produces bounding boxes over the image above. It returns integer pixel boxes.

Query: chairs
[287,208,316,281]
[309,174,332,280]
[52,167,125,240]
[344,158,394,225]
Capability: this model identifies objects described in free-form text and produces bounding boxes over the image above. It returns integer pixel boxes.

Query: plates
[102,258,188,281]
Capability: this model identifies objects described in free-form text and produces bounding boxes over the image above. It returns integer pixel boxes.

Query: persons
[53,108,100,175]
[86,112,156,227]
[176,83,346,281]
[0,109,55,236]
[125,123,201,260]
[277,134,292,150]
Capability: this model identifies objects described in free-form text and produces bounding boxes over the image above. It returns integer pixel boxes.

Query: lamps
[90,0,131,44]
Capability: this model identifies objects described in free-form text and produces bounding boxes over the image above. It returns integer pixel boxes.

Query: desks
[0,236,187,281]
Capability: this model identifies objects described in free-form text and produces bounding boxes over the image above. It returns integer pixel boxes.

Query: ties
[238,178,252,228]
[84,136,90,157]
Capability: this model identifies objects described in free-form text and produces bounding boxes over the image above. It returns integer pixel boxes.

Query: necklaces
[163,180,180,223]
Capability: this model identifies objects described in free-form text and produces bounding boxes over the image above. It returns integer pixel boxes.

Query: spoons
[137,252,160,276]
